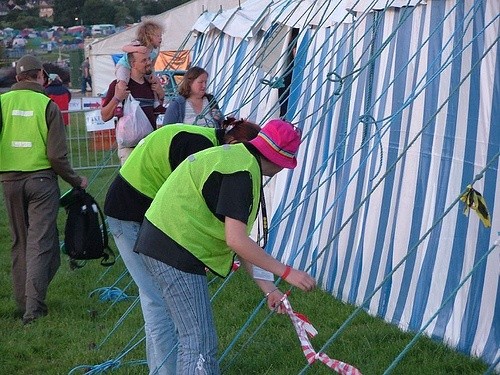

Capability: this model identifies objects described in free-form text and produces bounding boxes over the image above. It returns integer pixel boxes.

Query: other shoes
[154,105,167,114]
[19,304,47,325]
[114,104,123,117]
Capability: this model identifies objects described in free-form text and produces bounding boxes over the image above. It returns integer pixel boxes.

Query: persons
[113,22,167,116]
[132,119,317,375]
[101,45,165,167]
[103,116,262,375]
[41,56,92,128]
[163,66,225,129]
[0,55,89,328]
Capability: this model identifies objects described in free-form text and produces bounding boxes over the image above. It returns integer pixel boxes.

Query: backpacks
[64,189,115,269]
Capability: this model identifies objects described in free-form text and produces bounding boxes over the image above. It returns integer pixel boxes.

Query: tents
[68,0,500,375]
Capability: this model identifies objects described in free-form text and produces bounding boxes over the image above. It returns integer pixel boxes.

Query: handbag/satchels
[116,92,153,148]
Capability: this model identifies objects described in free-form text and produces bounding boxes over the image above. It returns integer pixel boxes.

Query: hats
[247,120,301,168]
[16,55,50,78]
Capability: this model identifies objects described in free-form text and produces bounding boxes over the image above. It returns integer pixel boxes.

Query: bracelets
[112,96,121,104]
[264,287,277,298]
[281,264,291,280]
